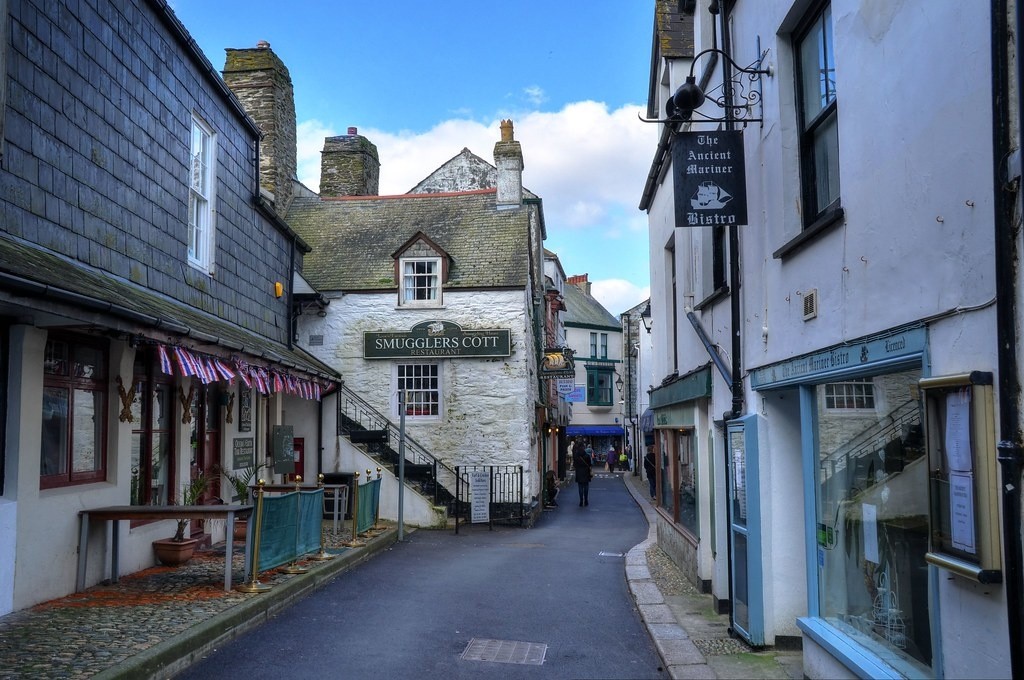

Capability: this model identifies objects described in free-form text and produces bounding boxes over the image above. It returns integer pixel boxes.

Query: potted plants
[152,471,221,564]
[213,461,270,540]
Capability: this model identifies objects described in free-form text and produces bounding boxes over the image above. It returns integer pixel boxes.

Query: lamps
[546,422,552,439]
[665,95,753,135]
[555,426,559,436]
[615,375,623,391]
[641,302,651,334]
[672,50,774,110]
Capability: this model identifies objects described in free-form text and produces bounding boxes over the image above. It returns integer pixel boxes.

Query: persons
[644,445,657,500]
[573,448,592,506]
[566,439,632,474]
[545,470,560,507]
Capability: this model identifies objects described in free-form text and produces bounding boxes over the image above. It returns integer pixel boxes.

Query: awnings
[566,425,625,436]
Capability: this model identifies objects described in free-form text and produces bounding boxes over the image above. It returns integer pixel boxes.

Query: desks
[77,505,254,593]
[244,484,347,536]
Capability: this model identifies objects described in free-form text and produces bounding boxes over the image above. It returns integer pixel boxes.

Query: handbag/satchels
[592,452,594,459]
[605,462,609,471]
